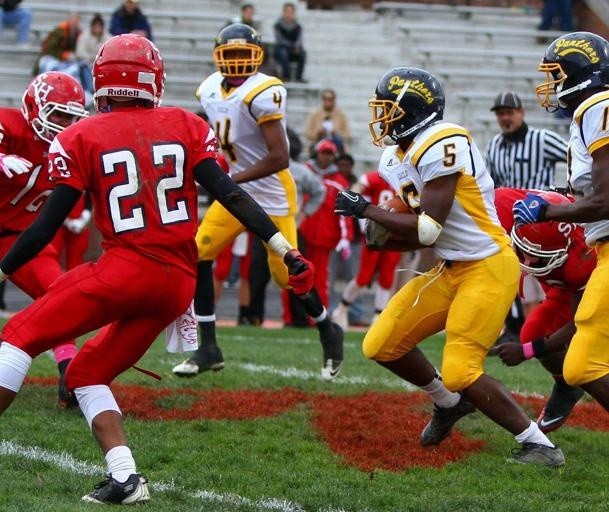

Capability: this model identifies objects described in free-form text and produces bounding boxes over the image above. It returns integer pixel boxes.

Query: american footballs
[376,198,416,251]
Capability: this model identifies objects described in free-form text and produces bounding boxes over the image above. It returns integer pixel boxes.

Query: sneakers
[81,473,151,505]
[57,378,77,410]
[536,381,584,432]
[421,392,474,445]
[172,302,385,382]
[507,442,566,469]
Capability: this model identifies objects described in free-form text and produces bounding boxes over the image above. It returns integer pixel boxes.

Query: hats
[490,92,521,111]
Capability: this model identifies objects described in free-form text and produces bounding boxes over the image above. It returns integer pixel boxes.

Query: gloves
[282,249,316,295]
[333,187,372,219]
[335,239,352,263]
[512,193,550,231]
[0,153,34,179]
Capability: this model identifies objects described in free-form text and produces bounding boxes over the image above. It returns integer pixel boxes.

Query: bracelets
[522,340,532,361]
[533,339,546,358]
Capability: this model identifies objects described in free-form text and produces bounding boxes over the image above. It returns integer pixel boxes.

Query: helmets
[212,23,265,78]
[510,192,575,276]
[368,67,445,147]
[535,32,609,113]
[20,72,89,145]
[93,33,165,112]
[313,140,338,155]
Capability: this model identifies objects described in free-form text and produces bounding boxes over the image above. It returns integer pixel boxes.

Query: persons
[511,31,609,416]
[0,32,320,505]
[481,91,568,330]
[214,3,414,329]
[493,191,598,434]
[172,22,346,383]
[0,0,153,111]
[0,72,92,412]
[332,65,568,471]
